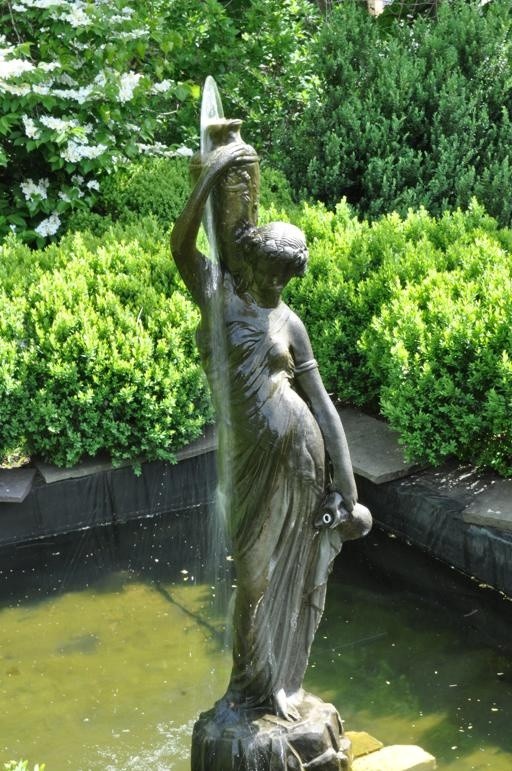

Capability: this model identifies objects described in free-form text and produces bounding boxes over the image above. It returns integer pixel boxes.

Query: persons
[170,142,359,736]
